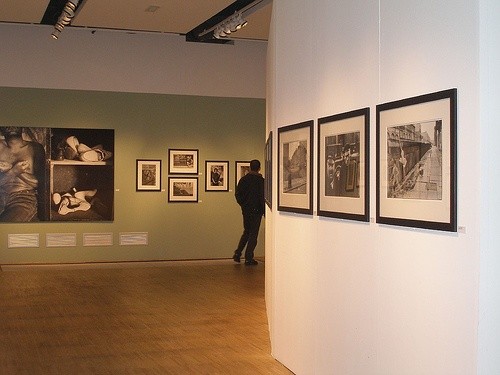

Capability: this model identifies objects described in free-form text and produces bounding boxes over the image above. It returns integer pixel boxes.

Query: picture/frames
[277,119,314,216]
[167,148,200,176]
[168,177,199,203]
[263,130,273,209]
[135,159,162,193]
[235,160,252,187]
[205,160,229,192]
[317,107,371,222]
[375,87,459,232]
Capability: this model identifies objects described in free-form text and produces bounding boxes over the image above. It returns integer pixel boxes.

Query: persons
[233,160,265,265]
[0,127,46,223]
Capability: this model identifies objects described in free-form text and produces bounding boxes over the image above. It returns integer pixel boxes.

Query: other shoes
[245,259,258,265]
[234,257,240,262]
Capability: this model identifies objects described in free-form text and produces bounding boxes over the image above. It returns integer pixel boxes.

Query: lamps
[50,0,79,40]
[214,11,248,39]
